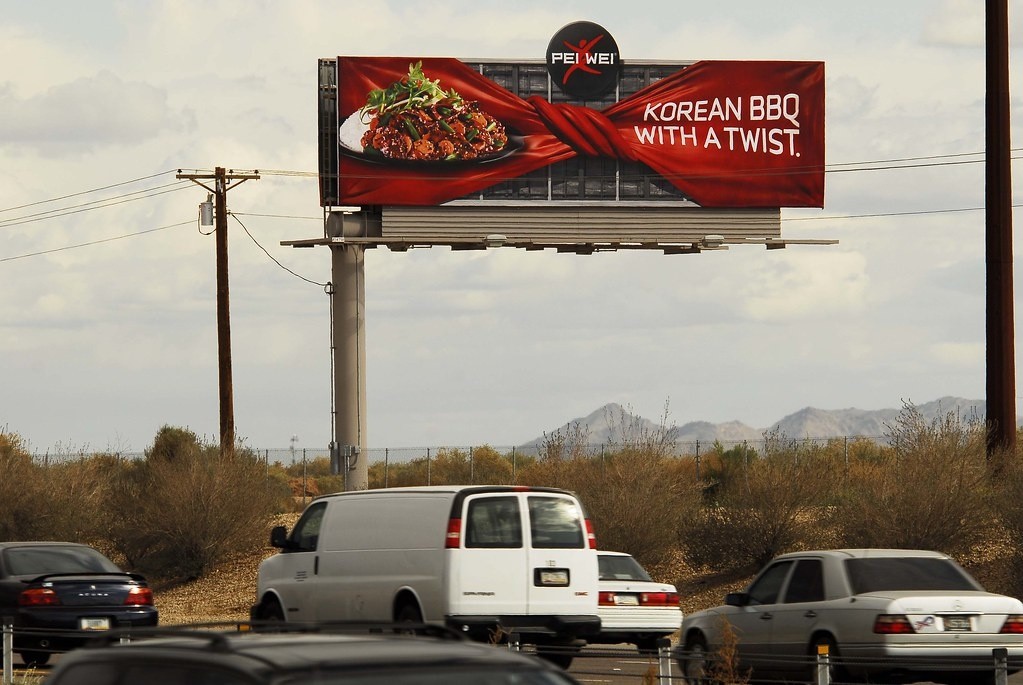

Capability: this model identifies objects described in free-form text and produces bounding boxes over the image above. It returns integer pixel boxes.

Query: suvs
[38,621,581,684]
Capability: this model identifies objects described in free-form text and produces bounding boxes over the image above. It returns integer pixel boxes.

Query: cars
[0,541,160,667]
[596,551,683,655]
[675,549,1022,684]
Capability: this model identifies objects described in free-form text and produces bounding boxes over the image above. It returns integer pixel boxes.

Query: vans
[248,484,602,669]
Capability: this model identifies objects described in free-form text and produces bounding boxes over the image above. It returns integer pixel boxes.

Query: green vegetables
[360,59,459,124]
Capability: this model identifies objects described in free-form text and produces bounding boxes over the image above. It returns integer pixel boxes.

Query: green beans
[363,106,504,159]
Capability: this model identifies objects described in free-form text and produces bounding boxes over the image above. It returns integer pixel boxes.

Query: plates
[340,121,522,170]
